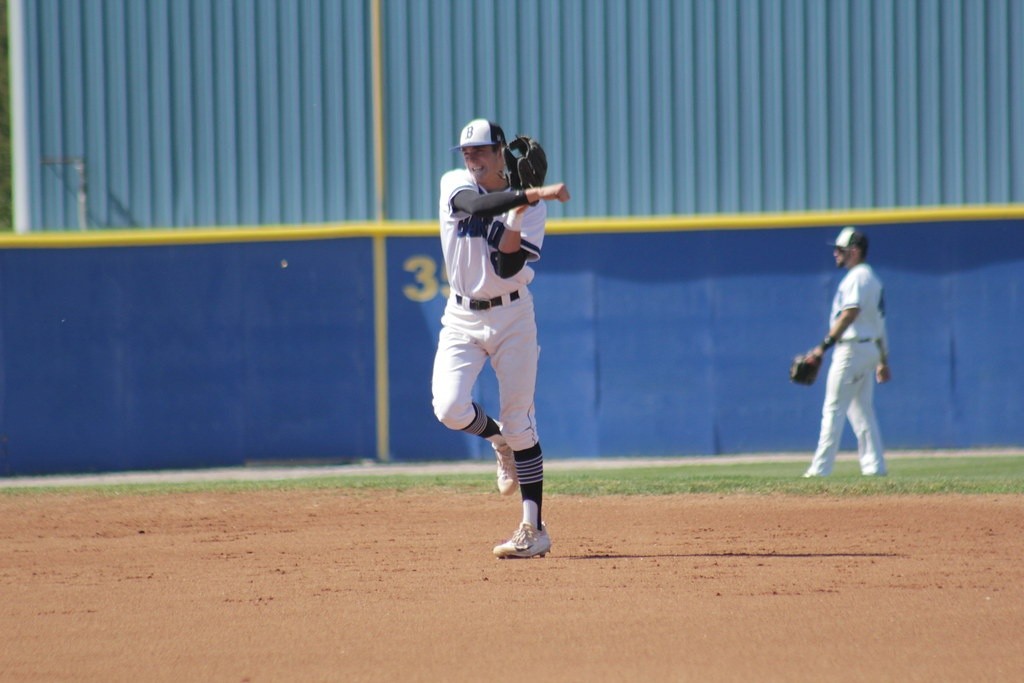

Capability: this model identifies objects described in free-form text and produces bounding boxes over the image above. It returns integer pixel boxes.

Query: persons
[430,117,571,558]
[789,226,890,478]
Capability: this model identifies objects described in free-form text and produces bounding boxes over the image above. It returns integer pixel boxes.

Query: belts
[456,290,519,310]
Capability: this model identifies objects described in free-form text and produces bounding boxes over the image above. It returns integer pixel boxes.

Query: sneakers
[484,418,520,496]
[493,521,551,560]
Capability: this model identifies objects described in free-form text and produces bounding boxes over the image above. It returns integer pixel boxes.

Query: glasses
[836,247,845,254]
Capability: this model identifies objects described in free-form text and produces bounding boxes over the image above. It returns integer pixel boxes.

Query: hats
[827,227,869,255]
[448,119,507,152]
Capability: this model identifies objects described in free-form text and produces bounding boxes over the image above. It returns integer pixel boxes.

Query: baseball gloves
[501,135,549,207]
[789,353,822,386]
